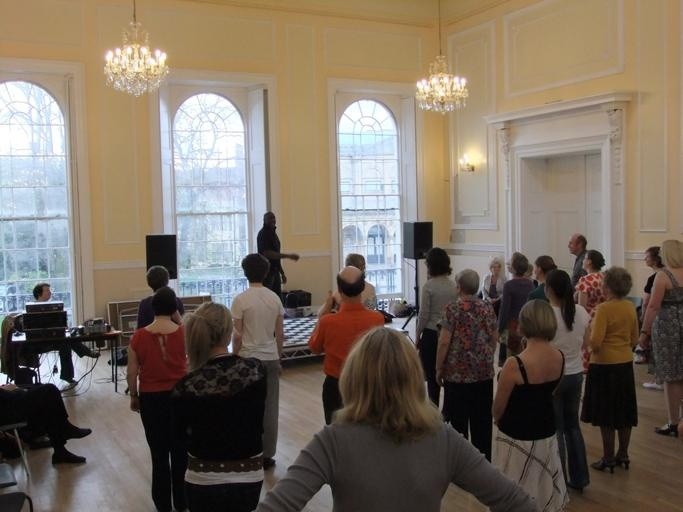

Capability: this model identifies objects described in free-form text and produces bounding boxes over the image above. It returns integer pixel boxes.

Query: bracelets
[640,330,654,336]
[130,392,138,396]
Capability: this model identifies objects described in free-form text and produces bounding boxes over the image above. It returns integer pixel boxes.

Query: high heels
[654,423,680,438]
[590,456,616,474]
[614,451,632,469]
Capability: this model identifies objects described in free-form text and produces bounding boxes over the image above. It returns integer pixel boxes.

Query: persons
[309,266,383,426]
[480,256,507,316]
[138,266,183,328]
[582,268,640,472]
[566,235,589,302]
[172,303,266,512]
[232,254,285,469]
[645,240,683,437]
[126,290,190,510]
[28,283,100,386]
[416,248,463,408]
[317,253,377,318]
[492,300,569,511]
[253,329,538,511]
[535,269,591,491]
[434,269,498,463]
[1,383,92,465]
[528,256,558,306]
[256,212,299,307]
[498,254,536,388]
[570,251,607,372]
[641,248,664,390]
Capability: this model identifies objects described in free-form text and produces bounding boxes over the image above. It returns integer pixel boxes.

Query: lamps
[103,0,170,98]
[458,148,480,172]
[415,1,469,115]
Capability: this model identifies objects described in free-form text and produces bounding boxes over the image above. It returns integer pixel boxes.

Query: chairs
[1,420,30,478]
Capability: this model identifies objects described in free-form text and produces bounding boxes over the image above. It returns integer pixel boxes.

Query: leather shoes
[52,423,93,444]
[59,375,79,385]
[86,348,103,360]
[50,450,87,466]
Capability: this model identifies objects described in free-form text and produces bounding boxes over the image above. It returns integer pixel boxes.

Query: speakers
[146,234,177,280]
[403,222,433,260]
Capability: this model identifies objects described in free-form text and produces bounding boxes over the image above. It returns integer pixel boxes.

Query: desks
[12,322,120,392]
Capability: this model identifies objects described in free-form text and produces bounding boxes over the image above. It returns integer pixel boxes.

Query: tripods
[401,260,419,330]
